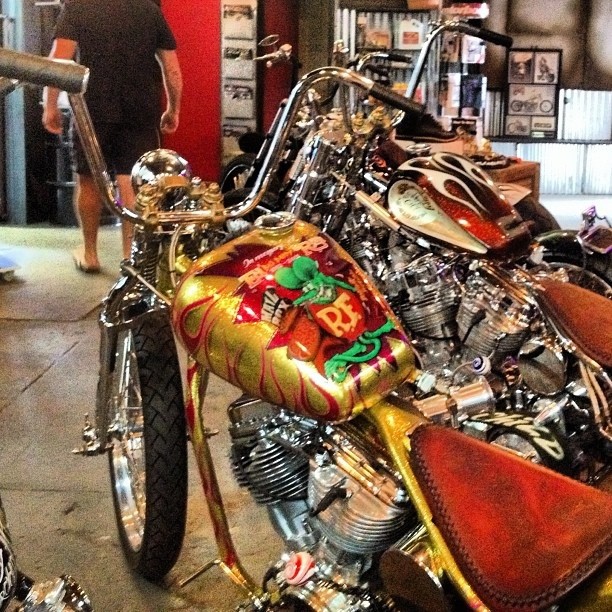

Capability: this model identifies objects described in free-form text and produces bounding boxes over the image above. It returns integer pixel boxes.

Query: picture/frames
[533,47,562,85]
[504,82,559,116]
[531,115,556,132]
[505,48,533,84]
[503,115,532,137]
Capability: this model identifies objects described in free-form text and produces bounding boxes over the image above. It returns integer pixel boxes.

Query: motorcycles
[0,50,611,611]
[218,19,612,612]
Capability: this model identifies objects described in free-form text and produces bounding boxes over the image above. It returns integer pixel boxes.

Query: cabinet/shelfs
[488,160,539,203]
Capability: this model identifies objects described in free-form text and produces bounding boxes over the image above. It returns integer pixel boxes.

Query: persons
[42,1,182,272]
